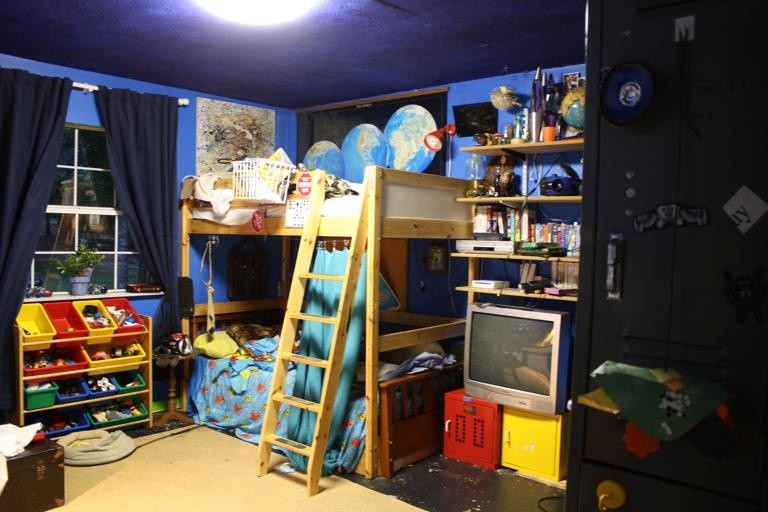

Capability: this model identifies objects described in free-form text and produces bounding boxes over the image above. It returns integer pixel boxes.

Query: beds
[181,165,473,480]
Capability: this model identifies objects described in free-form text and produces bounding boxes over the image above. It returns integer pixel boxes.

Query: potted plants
[56,243,106,295]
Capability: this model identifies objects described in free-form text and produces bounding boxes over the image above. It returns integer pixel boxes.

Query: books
[519,261,579,297]
[471,204,580,256]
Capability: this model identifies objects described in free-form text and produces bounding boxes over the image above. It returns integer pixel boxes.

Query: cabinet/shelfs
[12,314,153,440]
[443,388,503,470]
[501,405,572,482]
[449,138,585,304]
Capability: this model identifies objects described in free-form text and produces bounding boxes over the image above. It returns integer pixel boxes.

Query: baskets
[232,160,296,204]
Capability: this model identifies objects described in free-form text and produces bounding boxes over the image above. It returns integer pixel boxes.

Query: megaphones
[599,60,656,127]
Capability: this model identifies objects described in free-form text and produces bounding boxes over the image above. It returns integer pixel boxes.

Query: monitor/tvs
[464,303,575,415]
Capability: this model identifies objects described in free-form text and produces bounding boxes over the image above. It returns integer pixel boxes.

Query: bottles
[485,167,509,197]
[465,151,485,198]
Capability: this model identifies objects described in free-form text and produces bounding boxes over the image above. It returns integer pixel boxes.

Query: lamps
[423,125,457,178]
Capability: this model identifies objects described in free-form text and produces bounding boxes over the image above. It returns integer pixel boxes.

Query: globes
[560,87,586,138]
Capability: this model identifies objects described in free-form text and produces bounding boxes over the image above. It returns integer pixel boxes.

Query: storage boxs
[14,298,148,438]
[0,436,64,512]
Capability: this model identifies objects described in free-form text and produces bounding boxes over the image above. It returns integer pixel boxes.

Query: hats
[0,421,44,455]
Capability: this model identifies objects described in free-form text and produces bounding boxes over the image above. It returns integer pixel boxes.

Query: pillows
[194,325,272,357]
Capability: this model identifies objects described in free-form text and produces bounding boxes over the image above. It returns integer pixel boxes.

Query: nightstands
[153,354,194,426]
